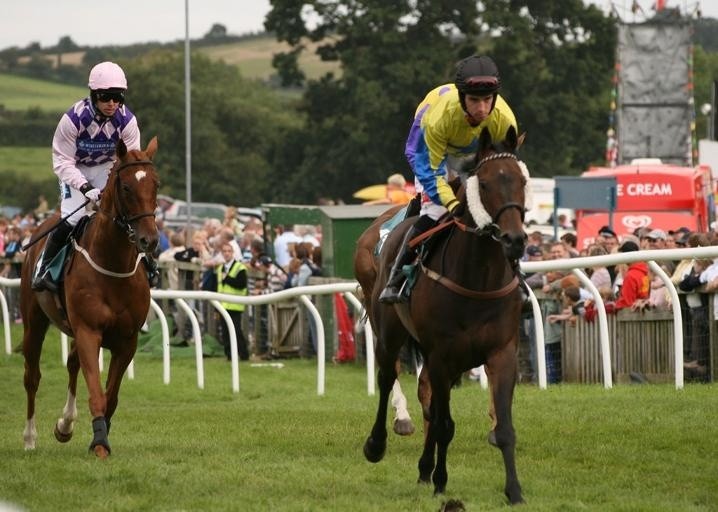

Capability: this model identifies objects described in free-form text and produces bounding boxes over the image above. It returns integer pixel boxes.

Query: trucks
[577,161,717,259]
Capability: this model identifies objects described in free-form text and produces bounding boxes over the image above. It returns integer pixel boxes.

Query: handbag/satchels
[301,259,322,277]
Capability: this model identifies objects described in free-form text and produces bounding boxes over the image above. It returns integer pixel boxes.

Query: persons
[1,195,48,324]
[158,205,319,360]
[31,61,158,292]
[364,174,415,207]
[519,214,717,382]
[377,56,529,304]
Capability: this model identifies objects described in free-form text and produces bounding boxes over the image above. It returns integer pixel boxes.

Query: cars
[163,201,228,234]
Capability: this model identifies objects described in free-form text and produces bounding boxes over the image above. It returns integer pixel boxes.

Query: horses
[354,123,528,508]
[20,134,162,465]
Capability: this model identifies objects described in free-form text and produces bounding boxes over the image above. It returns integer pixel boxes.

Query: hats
[526,245,543,255]
[598,225,696,251]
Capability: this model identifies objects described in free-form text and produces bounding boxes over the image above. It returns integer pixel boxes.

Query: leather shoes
[683,360,707,377]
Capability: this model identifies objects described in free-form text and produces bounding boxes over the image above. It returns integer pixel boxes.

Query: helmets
[87,61,128,91]
[454,54,501,95]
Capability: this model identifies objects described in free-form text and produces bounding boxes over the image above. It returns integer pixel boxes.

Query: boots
[518,284,533,319]
[377,224,434,304]
[31,231,70,292]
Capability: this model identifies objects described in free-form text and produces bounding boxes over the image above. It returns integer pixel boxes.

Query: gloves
[80,183,101,204]
[451,202,466,217]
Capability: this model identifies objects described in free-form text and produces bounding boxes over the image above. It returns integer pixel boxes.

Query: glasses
[94,91,125,102]
